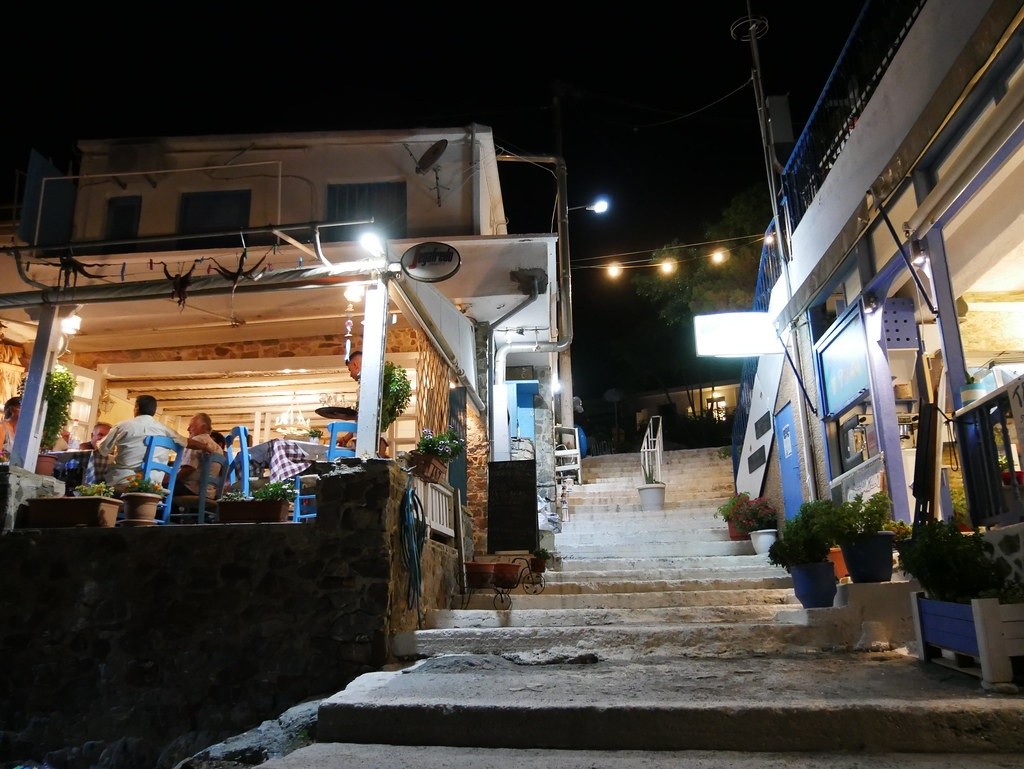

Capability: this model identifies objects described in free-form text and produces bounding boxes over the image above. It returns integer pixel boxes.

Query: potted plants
[15,364,78,475]
[637,464,667,511]
[897,511,1024,683]
[216,479,296,522]
[307,429,323,443]
[530,547,550,572]
[769,490,897,609]
[713,493,751,540]
[379,361,412,459]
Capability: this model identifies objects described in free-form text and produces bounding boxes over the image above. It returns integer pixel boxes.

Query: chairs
[170,453,228,523]
[226,425,278,500]
[121,436,185,525]
[294,422,358,522]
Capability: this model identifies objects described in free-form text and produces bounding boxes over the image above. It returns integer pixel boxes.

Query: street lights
[558,196,610,479]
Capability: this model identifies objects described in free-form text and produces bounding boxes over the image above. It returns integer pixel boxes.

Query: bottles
[2,428,12,462]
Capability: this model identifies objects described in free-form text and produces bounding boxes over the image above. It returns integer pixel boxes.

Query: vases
[121,493,161,527]
[25,496,124,527]
[496,563,521,589]
[462,561,493,587]
[750,529,777,555]
[410,453,447,483]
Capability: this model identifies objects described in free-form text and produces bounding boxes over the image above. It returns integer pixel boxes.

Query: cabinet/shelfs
[554,424,582,485]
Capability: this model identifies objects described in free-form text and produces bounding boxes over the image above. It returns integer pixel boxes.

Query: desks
[48,450,97,495]
[235,438,357,488]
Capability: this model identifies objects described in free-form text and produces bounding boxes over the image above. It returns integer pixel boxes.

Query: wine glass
[319,392,346,407]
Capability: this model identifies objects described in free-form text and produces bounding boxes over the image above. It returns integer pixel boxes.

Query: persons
[336,350,389,459]
[0,397,23,465]
[210,429,228,479]
[164,413,225,500]
[81,421,116,485]
[95,395,216,501]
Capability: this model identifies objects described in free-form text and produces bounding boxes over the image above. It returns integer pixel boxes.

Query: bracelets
[342,436,348,444]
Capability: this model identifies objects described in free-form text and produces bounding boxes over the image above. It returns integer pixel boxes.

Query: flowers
[122,471,165,494]
[416,425,466,463]
[76,482,116,497]
[731,497,778,533]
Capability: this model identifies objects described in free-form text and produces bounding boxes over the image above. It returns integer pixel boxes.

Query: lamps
[273,391,311,436]
